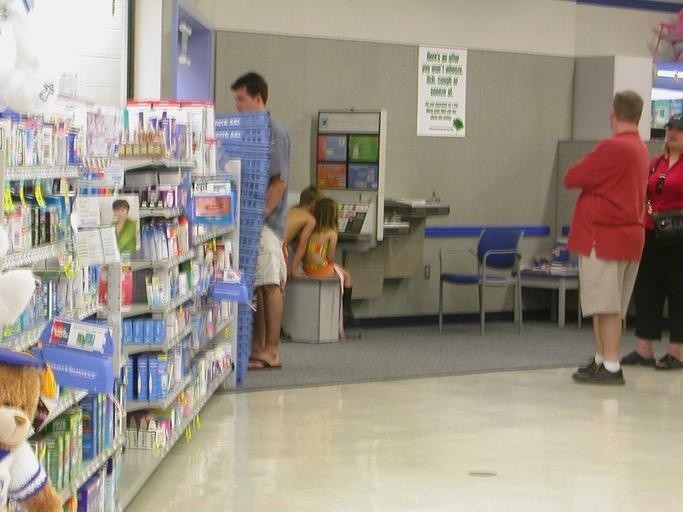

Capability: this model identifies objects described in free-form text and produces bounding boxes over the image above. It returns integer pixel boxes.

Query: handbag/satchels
[652,209,683,240]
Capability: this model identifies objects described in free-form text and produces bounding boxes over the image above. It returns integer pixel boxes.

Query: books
[519,262,576,277]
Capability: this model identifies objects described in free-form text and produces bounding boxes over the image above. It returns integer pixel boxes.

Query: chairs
[436,225,526,338]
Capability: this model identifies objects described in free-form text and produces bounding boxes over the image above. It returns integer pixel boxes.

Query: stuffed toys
[0,346,68,511]
[0,0,60,118]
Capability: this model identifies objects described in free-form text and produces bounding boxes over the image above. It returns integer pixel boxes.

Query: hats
[663,113,683,129]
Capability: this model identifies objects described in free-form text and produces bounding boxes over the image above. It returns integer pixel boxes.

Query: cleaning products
[553,225,576,262]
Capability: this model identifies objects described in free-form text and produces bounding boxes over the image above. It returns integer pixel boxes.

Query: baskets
[216,110,274,385]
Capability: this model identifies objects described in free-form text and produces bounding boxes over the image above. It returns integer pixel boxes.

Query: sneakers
[572,356,625,384]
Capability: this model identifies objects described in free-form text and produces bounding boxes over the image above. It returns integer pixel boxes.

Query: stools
[282,277,338,342]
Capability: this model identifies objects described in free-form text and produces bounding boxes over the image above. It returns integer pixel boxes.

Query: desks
[512,265,579,327]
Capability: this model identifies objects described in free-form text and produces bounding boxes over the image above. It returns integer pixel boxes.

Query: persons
[621,113,683,370]
[563,90,650,386]
[303,198,355,320]
[110,199,137,252]
[229,70,291,371]
[279,185,322,343]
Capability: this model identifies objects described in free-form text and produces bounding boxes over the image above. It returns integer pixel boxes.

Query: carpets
[214,323,683,395]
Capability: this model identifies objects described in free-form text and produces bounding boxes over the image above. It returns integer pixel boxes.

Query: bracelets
[263,204,273,216]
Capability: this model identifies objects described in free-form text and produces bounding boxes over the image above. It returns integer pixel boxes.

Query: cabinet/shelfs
[120,157,243,446]
[0,163,120,512]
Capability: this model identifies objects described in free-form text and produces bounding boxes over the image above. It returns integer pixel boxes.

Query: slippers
[654,353,683,369]
[621,351,656,366]
[248,358,282,370]
